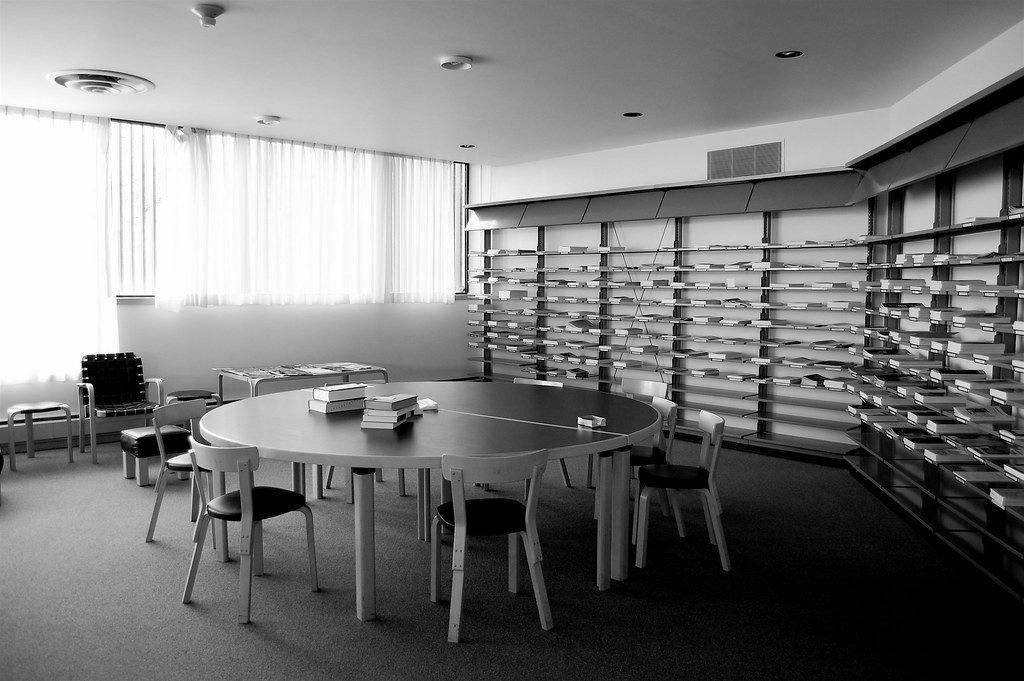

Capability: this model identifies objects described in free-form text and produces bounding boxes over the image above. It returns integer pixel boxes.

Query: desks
[219,363,389,403]
[198,381,663,621]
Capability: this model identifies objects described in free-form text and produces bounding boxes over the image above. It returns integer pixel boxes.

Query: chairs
[78,353,728,645]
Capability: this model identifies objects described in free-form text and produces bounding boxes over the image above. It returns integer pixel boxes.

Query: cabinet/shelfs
[465,210,1024,599]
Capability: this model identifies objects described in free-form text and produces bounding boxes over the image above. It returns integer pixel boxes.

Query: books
[308,381,419,430]
[467,203,1023,507]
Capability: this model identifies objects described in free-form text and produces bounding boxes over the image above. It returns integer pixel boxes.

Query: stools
[7,401,73,471]
[166,390,221,430]
